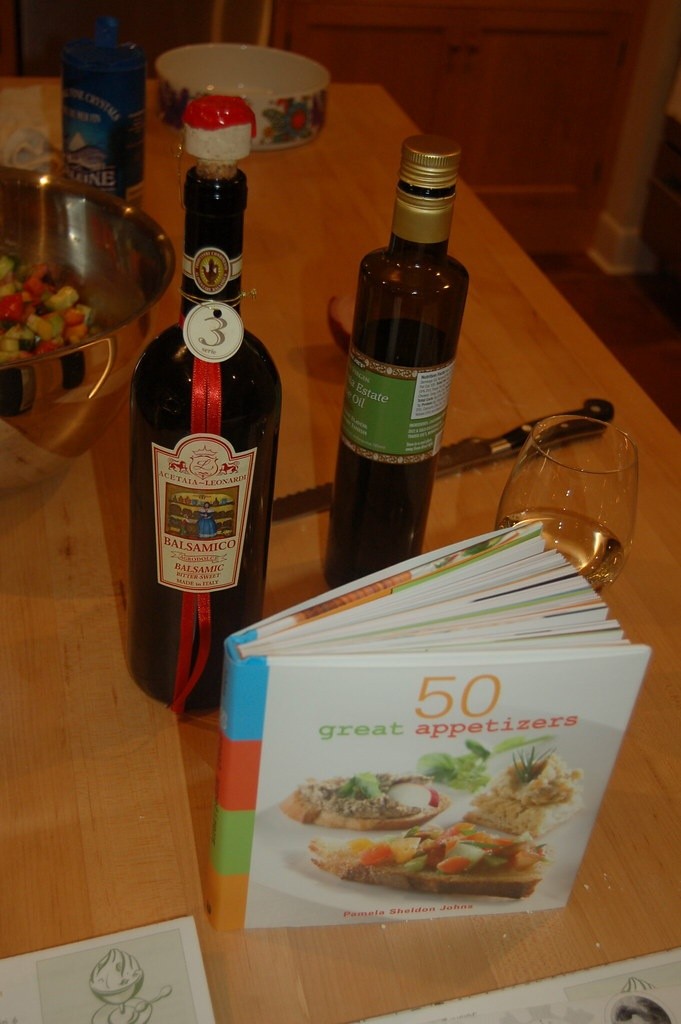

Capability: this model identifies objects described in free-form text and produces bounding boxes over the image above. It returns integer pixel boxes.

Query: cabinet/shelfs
[268,0,647,257]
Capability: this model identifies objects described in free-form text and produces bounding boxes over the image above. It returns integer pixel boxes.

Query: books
[203,518,653,932]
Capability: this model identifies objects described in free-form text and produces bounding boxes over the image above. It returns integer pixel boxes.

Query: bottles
[58,19,147,203]
[127,96,283,713]
[324,134,470,591]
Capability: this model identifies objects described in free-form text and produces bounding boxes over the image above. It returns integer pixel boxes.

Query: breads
[464,789,582,838]
[308,839,547,900]
[279,780,450,831]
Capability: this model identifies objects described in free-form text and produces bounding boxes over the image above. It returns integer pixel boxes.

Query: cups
[495,415,640,593]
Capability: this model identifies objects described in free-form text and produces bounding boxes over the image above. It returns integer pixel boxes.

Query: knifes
[270,398,616,524]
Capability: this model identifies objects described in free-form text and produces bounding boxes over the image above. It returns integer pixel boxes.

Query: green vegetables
[417,734,556,792]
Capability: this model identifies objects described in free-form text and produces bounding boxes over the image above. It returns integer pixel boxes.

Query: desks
[1,84,681,1024]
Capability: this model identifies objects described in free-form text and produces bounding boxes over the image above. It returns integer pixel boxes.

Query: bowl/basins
[0,164,175,487]
